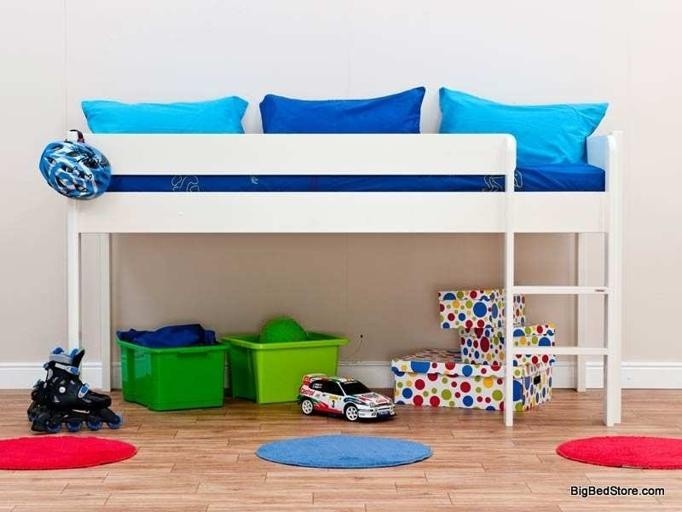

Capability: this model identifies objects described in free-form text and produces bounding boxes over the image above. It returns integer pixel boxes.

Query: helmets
[35,140,111,200]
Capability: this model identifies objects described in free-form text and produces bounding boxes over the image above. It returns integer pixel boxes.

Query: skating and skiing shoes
[26,345,122,433]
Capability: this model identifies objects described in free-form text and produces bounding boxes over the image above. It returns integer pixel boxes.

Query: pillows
[259,87,426,133]
[82,96,249,135]
[437,88,609,166]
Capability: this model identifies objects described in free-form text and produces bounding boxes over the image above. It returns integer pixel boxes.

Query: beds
[68,131,623,427]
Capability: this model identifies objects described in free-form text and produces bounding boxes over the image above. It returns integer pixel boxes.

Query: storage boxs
[437,288,526,329]
[459,320,558,365]
[391,346,558,412]
[222,330,351,405]
[114,332,229,412]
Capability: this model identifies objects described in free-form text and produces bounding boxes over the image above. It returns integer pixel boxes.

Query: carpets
[0,435,137,470]
[254,435,432,469]
[556,435,682,470]
